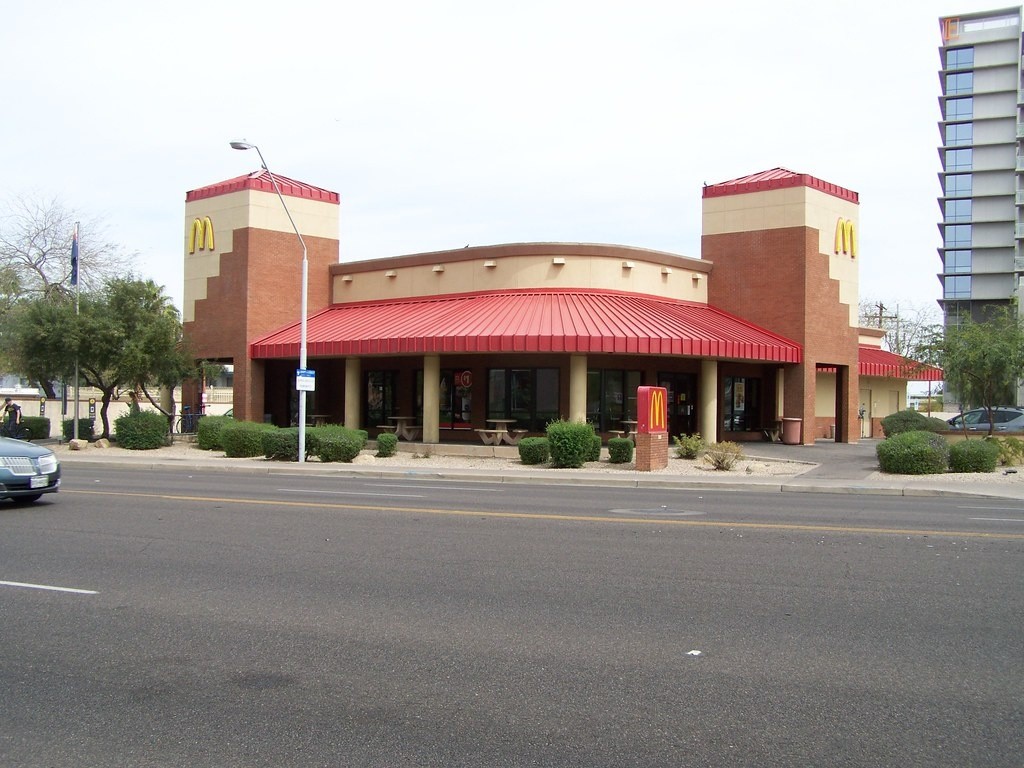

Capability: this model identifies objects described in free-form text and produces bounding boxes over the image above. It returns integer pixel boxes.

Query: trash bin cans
[782,417,803,445]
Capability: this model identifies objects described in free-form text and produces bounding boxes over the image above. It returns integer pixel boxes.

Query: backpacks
[7,406,21,418]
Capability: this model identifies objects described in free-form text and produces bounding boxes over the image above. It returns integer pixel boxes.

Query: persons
[126,391,140,414]
[0,397,21,438]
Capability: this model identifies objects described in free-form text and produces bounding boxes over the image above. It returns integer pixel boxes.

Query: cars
[946,405,1024,431]
[0,436,61,503]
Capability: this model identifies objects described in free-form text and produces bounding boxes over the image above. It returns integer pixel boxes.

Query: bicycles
[0,421,33,442]
[176,403,211,434]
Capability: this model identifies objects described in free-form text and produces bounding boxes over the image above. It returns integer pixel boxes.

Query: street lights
[229,141,318,463]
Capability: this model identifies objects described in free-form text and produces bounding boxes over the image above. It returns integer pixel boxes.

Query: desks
[486,419,517,446]
[307,415,334,427]
[387,417,416,441]
[769,420,783,442]
[620,421,638,441]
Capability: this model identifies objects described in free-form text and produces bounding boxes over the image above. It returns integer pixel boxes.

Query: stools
[474,429,528,446]
[755,428,783,442]
[608,430,637,442]
[306,423,327,427]
[376,426,422,441]
